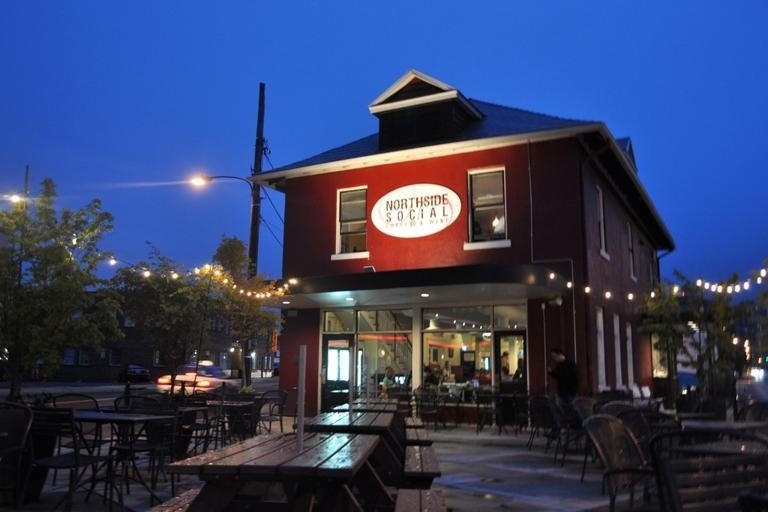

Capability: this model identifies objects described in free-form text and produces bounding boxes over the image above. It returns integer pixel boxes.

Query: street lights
[9,164,30,401]
[187,83,265,379]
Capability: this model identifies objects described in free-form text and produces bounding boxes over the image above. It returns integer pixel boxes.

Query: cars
[119,365,151,383]
[658,372,701,412]
[157,361,241,395]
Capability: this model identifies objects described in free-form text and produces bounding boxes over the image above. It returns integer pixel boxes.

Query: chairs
[0,369,768,511]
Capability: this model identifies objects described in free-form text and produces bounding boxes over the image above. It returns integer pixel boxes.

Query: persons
[501,351,514,383]
[440,359,452,377]
[469,368,491,404]
[543,345,589,440]
[381,366,399,398]
[425,365,438,386]
